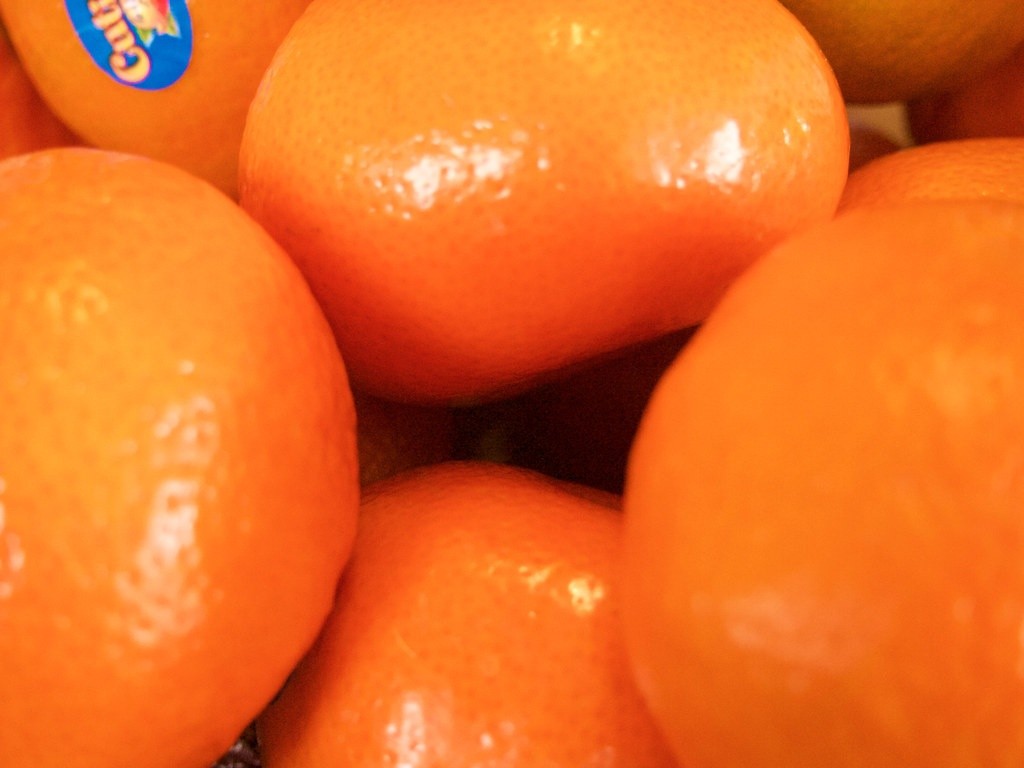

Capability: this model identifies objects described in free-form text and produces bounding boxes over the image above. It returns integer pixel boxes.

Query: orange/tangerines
[0,0,1024,768]
